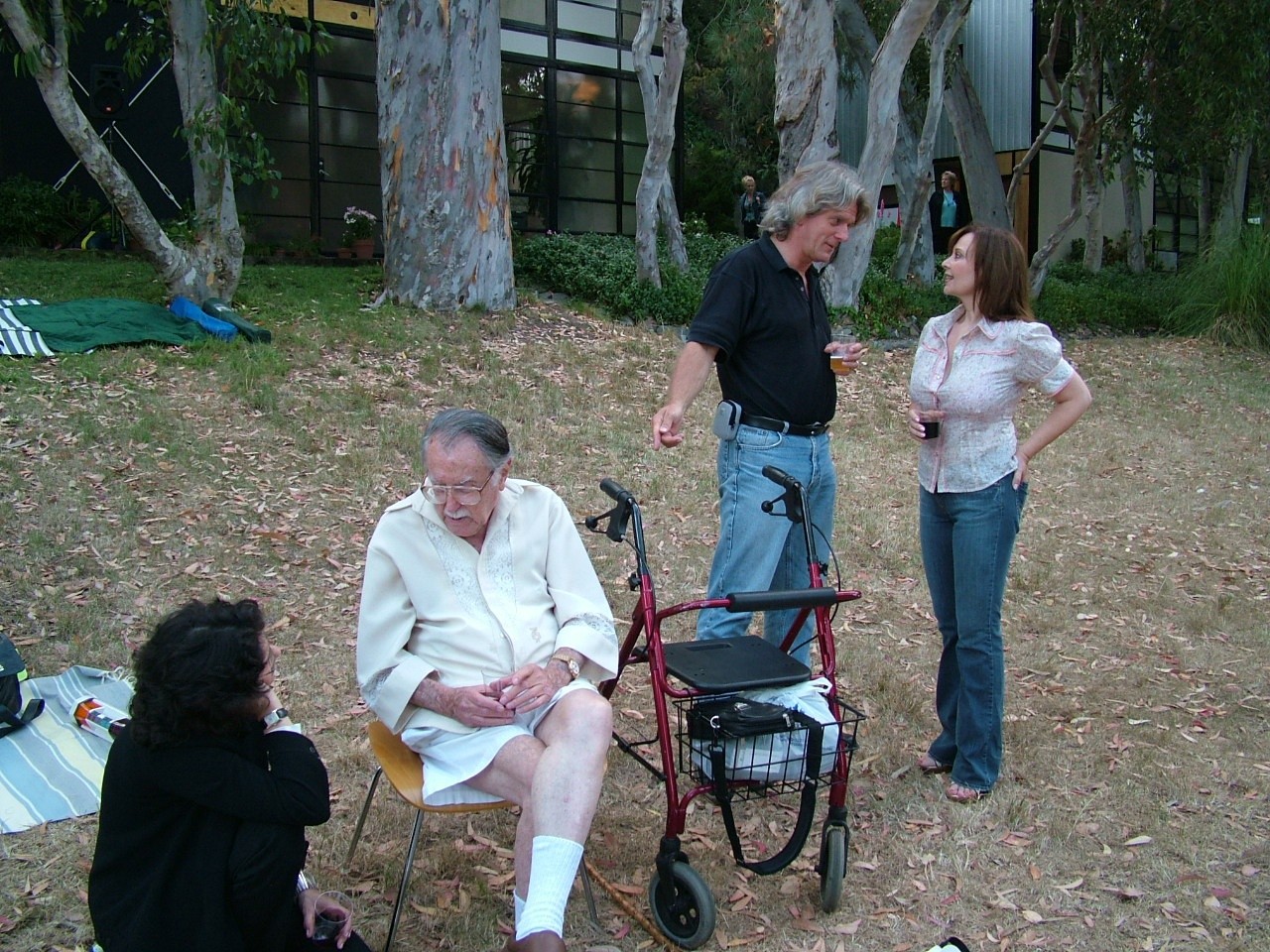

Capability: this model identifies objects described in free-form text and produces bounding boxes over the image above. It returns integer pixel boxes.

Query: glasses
[421,464,500,506]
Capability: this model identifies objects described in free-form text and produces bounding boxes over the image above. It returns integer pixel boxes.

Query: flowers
[339,206,377,248]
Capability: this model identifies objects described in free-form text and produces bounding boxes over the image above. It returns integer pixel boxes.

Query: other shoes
[508,929,567,952]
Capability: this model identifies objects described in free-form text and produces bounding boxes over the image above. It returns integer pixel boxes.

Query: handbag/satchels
[0,630,45,738]
[690,679,841,782]
[686,698,795,737]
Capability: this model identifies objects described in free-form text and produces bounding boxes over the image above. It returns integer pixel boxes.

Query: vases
[338,246,352,258]
[354,239,375,259]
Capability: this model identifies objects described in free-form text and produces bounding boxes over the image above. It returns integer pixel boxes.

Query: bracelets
[295,870,318,895]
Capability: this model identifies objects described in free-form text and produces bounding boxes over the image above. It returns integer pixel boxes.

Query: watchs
[260,707,288,731]
[550,653,580,681]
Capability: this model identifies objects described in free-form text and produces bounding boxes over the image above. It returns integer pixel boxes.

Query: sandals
[921,755,954,773]
[945,782,990,803]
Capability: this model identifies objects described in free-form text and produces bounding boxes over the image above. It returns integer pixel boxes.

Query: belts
[739,413,831,436]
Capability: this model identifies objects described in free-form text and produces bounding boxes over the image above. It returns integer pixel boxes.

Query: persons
[89,597,372,952]
[652,162,874,702]
[354,408,620,952]
[738,175,768,242]
[909,224,1093,804]
[928,170,973,256]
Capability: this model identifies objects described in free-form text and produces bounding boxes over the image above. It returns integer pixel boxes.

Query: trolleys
[585,465,873,952]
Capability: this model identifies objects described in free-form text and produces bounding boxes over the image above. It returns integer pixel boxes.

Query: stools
[340,720,598,952]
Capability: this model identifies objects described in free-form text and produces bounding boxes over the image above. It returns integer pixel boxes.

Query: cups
[312,890,354,939]
[481,663,518,716]
[917,400,941,439]
[831,333,856,374]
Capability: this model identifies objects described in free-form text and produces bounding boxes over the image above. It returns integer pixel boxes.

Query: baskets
[671,696,868,807]
